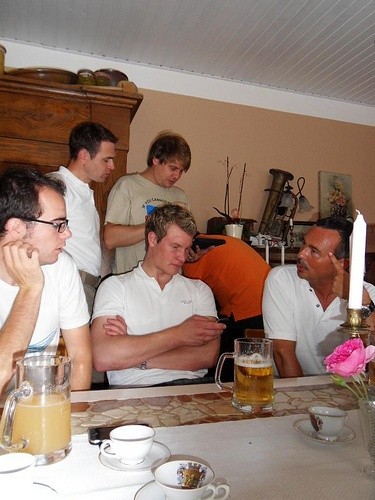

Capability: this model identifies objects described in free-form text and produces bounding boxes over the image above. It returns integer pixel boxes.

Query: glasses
[22,217,69,233]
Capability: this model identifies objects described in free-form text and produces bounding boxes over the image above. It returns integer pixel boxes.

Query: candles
[282,245,284,266]
[266,245,269,264]
[348,209,367,309]
[290,218,293,226]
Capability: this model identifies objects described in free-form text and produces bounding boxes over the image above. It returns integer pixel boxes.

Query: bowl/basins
[6,67,76,84]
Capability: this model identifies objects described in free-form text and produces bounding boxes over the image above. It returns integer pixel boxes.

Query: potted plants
[212,156,246,240]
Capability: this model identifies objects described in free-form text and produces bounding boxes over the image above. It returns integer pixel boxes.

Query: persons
[262,216,375,379]
[0,161,93,400]
[44,121,115,325]
[182,231,272,383]
[104,131,191,275]
[90,203,226,389]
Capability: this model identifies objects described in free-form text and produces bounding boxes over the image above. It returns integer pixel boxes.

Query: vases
[358,396,375,481]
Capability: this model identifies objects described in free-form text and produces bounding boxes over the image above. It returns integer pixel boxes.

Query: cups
[0,452,34,500]
[98,423,156,464]
[215,337,274,413]
[152,459,230,499]
[0,353,72,466]
[306,406,347,441]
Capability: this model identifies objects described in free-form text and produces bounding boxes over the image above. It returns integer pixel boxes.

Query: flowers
[323,338,375,410]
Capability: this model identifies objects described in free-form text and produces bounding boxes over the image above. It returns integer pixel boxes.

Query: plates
[134,480,219,500]
[34,483,59,500]
[291,417,356,445]
[98,439,169,472]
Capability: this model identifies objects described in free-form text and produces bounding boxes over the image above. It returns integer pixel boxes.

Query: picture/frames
[282,221,317,249]
[318,171,353,221]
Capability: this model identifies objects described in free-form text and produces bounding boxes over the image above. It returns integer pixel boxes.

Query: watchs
[361,300,375,319]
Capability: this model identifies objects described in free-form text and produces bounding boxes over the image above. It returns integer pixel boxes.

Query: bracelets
[141,360,146,369]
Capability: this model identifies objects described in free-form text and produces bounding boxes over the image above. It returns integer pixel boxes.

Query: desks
[0,375,375,500]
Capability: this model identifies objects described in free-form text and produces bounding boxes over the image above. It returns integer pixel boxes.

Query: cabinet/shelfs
[0,74,143,225]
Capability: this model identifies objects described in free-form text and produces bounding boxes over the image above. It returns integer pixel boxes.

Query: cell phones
[88,424,149,444]
[216,318,228,324]
